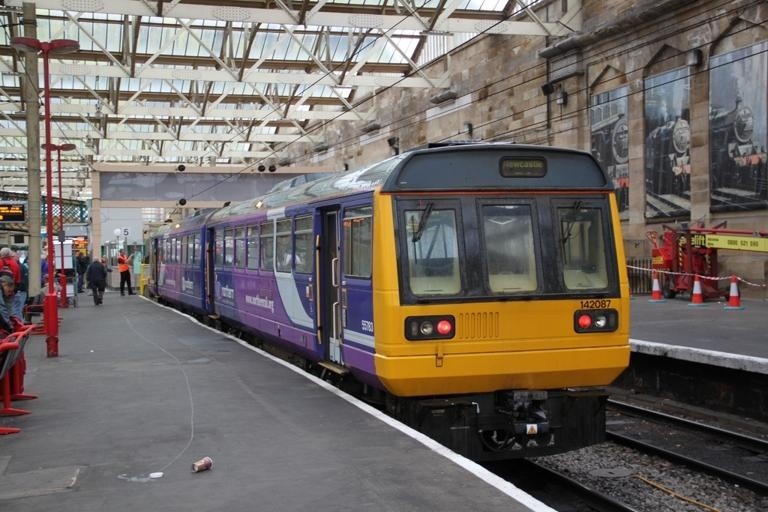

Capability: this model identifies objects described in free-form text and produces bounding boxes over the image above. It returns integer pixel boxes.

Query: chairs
[0,288,63,433]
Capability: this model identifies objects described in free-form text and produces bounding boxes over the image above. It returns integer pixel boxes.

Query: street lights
[9,37,80,356]
[41,144,76,308]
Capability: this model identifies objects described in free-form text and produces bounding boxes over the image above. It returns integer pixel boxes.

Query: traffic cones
[723,274,746,310]
[648,271,668,303]
[688,272,709,307]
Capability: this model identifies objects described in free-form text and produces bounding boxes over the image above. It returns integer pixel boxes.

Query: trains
[141,139,630,464]
[590,92,766,214]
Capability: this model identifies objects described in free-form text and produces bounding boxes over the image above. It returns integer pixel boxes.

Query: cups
[190,456,214,474]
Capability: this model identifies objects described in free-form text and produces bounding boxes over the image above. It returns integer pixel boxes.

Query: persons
[0,246,59,392]
[76,252,88,293]
[86,256,107,305]
[117,248,137,295]
